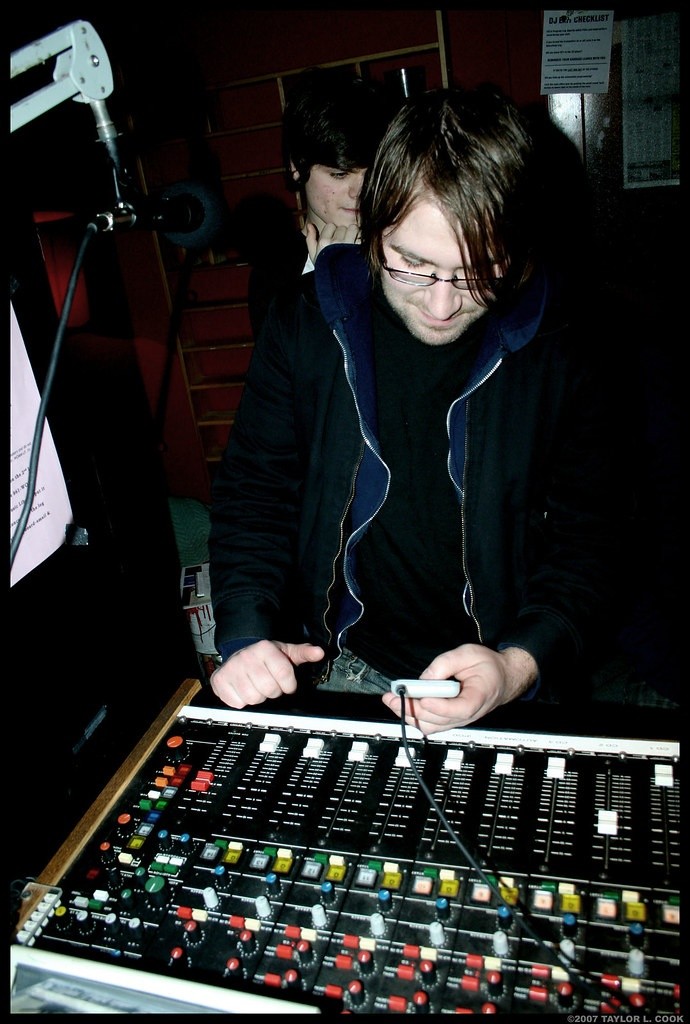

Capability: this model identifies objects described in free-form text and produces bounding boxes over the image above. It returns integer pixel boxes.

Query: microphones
[88,193,207,233]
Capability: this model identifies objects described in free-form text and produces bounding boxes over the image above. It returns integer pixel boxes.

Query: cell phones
[389,679,461,700]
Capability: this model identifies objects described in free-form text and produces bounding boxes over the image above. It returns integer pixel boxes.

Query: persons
[210,66,624,728]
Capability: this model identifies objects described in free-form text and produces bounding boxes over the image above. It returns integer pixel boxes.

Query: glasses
[376,233,515,290]
